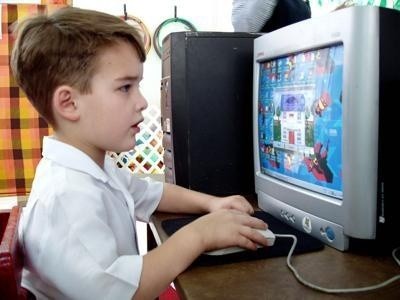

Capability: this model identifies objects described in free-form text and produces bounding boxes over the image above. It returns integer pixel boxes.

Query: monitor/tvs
[254,6,400,252]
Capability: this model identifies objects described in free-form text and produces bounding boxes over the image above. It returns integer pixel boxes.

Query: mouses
[204,228,275,256]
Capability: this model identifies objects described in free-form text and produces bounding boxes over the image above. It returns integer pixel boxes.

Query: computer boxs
[160,30,261,197]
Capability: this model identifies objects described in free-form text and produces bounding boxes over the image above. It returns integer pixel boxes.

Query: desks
[147,174,400,300]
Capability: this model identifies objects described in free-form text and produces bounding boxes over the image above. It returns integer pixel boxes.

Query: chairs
[0,205,30,300]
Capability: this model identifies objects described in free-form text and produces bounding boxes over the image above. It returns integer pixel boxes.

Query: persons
[10,6,270,300]
